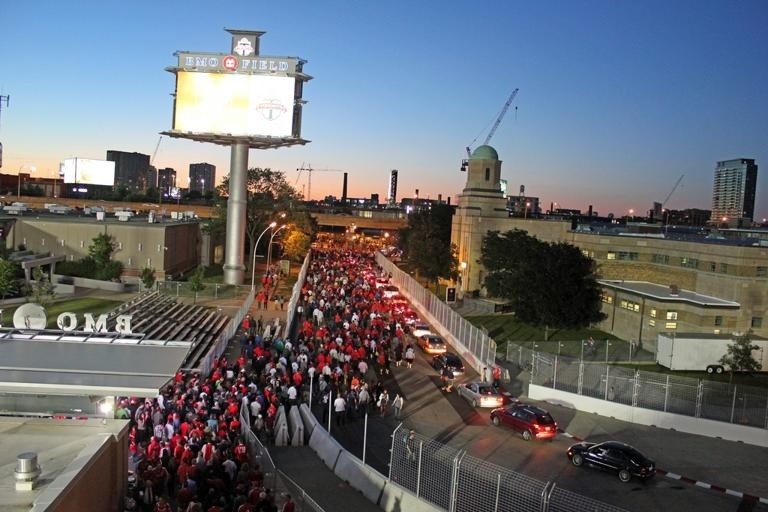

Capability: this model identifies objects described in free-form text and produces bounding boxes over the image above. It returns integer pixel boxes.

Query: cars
[566,441,656,483]
[490,402,556,442]
[433,352,464,376]
[457,382,503,408]
[364,268,447,354]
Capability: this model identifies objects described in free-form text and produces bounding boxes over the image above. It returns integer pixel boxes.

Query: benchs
[78,290,228,370]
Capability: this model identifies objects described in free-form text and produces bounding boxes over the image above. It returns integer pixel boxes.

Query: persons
[440,367,456,392]
[498,368,502,380]
[294,320,371,430]
[297,237,396,324]
[371,317,415,375]
[280,296,284,310]
[377,389,389,419]
[241,312,293,361]
[263,292,268,312]
[275,295,278,311]
[256,291,262,310]
[392,393,403,420]
[493,366,499,389]
[261,263,283,298]
[586,335,597,357]
[115,355,302,511]
[481,367,487,384]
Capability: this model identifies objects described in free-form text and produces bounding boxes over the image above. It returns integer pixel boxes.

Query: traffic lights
[447,287,457,304]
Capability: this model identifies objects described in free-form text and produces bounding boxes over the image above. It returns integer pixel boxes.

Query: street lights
[250,214,287,290]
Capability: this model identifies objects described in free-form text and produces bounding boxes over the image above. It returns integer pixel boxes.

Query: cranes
[296,162,343,200]
[460,88,519,172]
